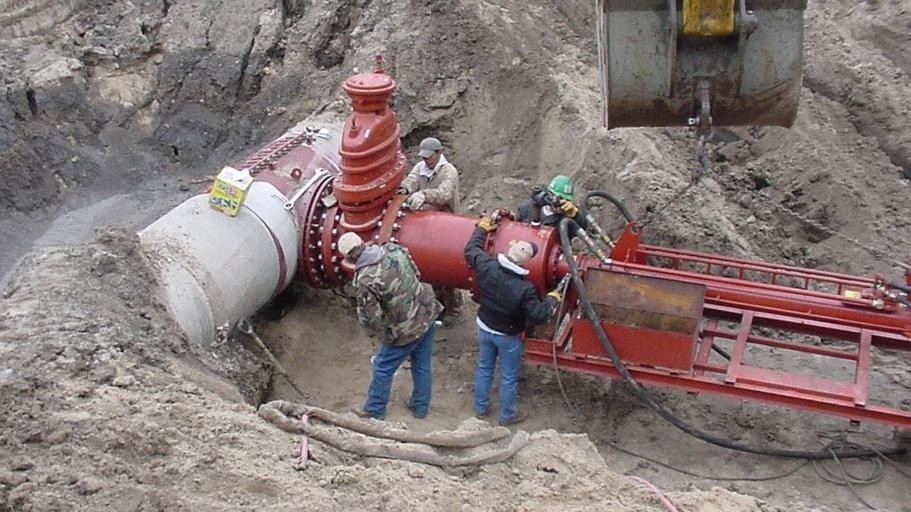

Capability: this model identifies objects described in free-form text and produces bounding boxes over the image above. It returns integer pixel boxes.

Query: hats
[338,231,362,258]
[417,137,443,159]
[509,241,538,263]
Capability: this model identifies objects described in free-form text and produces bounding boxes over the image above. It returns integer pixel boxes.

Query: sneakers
[480,404,496,418]
[404,396,411,408]
[351,406,372,417]
[500,410,528,426]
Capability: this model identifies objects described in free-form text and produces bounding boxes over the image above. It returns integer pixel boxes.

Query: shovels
[237,318,317,407]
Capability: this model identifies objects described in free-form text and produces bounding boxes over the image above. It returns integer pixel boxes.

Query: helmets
[548,175,575,201]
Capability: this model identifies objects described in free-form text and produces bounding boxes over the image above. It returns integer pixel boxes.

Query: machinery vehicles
[593,0,812,131]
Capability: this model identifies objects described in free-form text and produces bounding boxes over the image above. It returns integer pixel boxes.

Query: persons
[458,213,562,428]
[494,172,587,244]
[394,136,461,326]
[334,230,445,423]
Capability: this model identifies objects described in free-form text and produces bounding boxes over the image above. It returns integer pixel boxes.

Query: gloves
[478,216,497,232]
[406,191,425,211]
[548,292,563,301]
[560,199,578,217]
